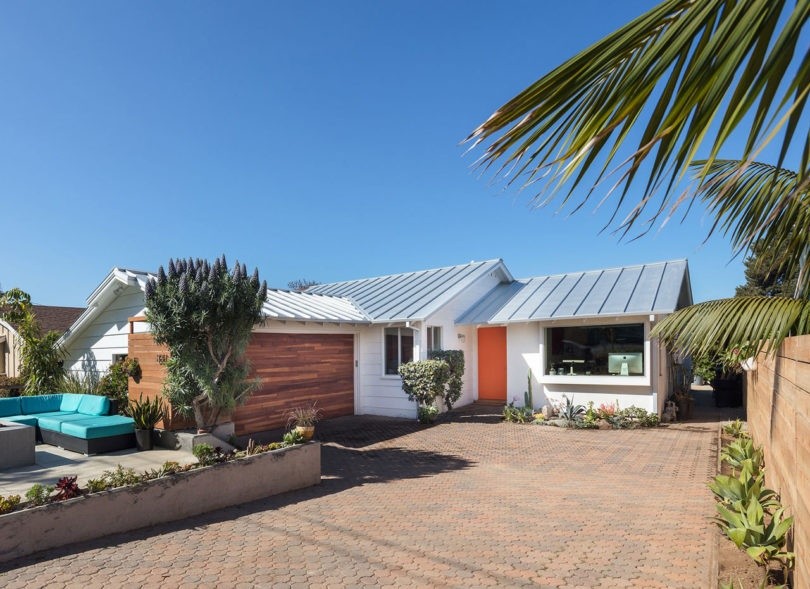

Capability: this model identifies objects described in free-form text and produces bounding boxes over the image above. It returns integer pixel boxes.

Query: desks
[0,421,35,470]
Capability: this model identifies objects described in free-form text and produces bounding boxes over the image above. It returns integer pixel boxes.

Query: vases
[740,360,750,371]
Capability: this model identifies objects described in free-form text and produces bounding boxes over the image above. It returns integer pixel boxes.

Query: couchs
[0,393,135,455]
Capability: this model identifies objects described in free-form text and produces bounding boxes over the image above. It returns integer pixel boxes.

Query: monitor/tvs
[608,352,642,376]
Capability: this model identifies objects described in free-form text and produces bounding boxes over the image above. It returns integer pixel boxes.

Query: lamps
[458,334,465,343]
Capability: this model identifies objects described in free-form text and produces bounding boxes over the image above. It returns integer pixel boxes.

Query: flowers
[718,344,743,373]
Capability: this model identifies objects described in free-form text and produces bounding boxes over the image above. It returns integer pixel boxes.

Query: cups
[558,368,564,375]
[549,371,556,374]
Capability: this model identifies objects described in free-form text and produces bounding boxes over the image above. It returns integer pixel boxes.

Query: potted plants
[130,392,163,451]
[281,400,325,439]
[742,345,758,370]
[121,359,137,376]
[97,373,120,415]
[674,363,696,420]
[558,394,586,429]
